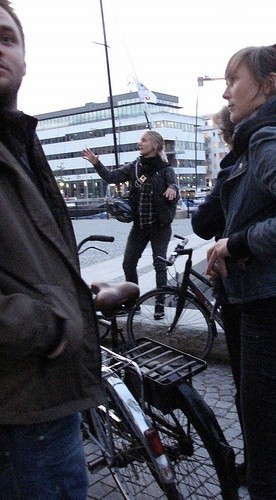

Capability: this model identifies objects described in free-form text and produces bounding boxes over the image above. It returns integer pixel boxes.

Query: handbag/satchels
[106,201,133,223]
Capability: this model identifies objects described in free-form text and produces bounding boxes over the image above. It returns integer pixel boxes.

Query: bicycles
[79,235,240,500]
[125,235,224,368]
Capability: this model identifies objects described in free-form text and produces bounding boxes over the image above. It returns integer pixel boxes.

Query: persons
[190,43,276,500]
[81,131,179,319]
[0,0,108,500]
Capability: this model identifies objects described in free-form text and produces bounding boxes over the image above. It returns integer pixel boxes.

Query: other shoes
[154,305,165,319]
[116,306,141,316]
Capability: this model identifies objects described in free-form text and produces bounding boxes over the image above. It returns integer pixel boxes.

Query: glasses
[218,132,227,146]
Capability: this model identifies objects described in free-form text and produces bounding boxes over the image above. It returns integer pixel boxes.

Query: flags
[136,82,157,104]
